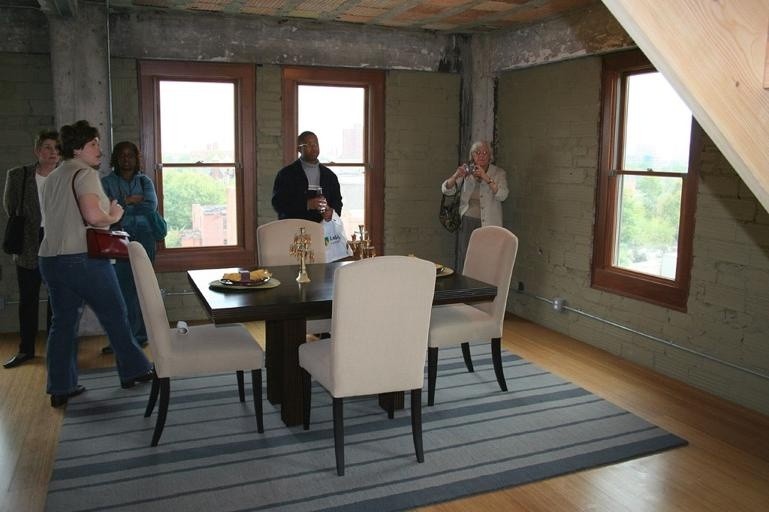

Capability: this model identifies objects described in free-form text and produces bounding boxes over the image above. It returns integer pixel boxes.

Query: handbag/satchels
[87,229,131,259]
[438,199,461,233]
[4,217,24,254]
[147,210,168,241]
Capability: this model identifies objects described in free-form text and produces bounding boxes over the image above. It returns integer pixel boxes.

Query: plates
[436,263,444,274]
[219,275,271,286]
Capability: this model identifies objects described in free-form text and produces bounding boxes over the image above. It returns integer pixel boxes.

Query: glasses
[298,142,318,150]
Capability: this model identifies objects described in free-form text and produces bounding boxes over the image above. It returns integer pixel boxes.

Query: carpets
[45,340,689,512]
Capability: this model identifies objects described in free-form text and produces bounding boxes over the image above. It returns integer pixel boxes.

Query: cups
[318,188,326,213]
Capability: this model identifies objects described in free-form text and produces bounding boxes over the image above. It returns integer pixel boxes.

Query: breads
[223,269,268,282]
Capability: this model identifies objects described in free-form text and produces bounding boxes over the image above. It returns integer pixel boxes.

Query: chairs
[427,226,519,406]
[297,255,437,476]
[258,217,325,264]
[128,241,266,447]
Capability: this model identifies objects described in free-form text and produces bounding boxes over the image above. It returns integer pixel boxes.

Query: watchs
[488,178,493,184]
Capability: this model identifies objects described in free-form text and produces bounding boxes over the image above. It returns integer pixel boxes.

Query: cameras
[464,161,476,174]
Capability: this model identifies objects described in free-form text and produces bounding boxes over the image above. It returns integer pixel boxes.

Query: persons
[101,141,158,354]
[3,131,60,369]
[38,120,154,407]
[271,131,342,223]
[442,142,511,273]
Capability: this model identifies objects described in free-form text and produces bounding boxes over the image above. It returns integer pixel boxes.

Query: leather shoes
[122,363,155,388]
[3,354,34,368]
[50,386,85,408]
